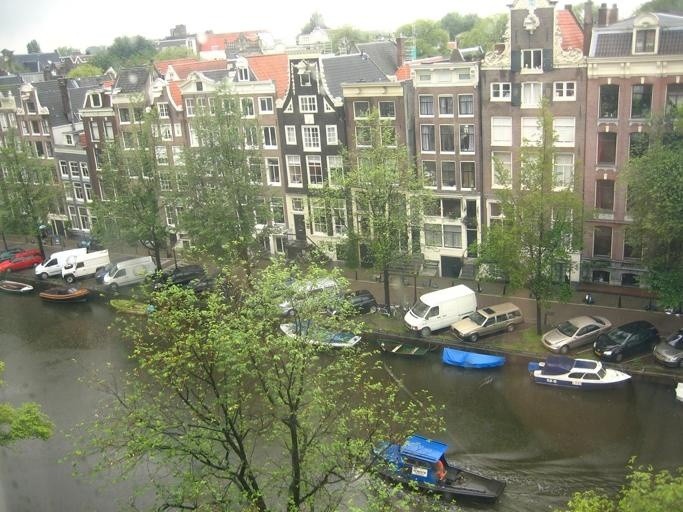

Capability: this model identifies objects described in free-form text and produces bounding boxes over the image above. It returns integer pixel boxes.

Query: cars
[651,330,682,369]
[541,314,612,356]
[591,320,657,362]
[323,291,378,319]
[0,248,42,273]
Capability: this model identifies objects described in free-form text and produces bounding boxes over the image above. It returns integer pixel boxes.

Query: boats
[276,322,361,350]
[439,346,506,370]
[0,279,37,294]
[674,382,682,402]
[523,356,633,391]
[109,297,161,318]
[376,338,426,359]
[39,286,89,302]
[359,435,505,505]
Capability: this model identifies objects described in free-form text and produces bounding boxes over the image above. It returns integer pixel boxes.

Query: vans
[402,283,476,338]
[33,246,156,291]
[276,277,338,319]
[449,302,524,343]
[152,264,205,292]
